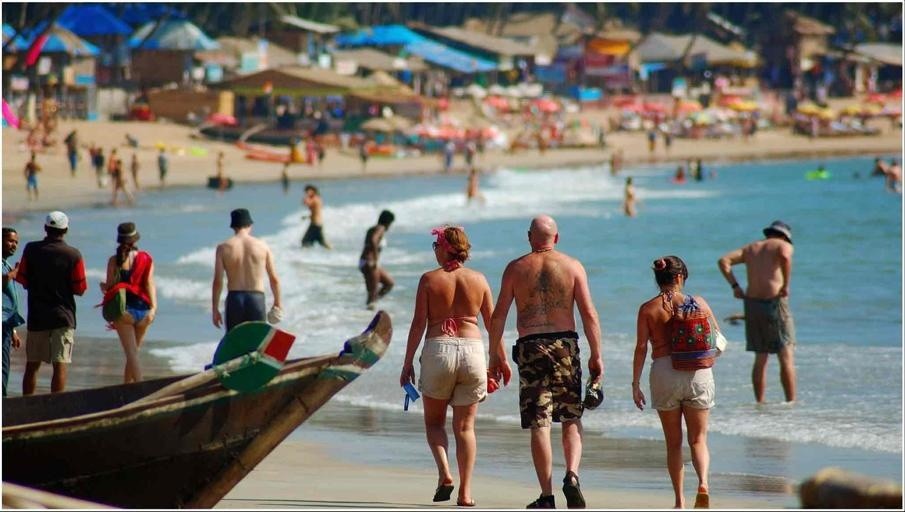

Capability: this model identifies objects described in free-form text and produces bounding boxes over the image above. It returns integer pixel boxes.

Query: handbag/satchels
[670,295,716,372]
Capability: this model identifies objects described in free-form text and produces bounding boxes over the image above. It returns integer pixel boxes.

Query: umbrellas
[2,18,102,58]
[56,0,132,36]
[122,12,221,53]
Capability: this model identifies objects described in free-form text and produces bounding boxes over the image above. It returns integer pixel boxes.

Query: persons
[632,255,728,510]
[107,148,118,174]
[870,159,888,176]
[717,220,798,406]
[112,160,133,203]
[689,157,698,177]
[131,154,140,189]
[125,133,139,147]
[17,209,88,396]
[359,208,396,310]
[400,224,511,507]
[443,137,456,169]
[158,147,167,182]
[64,130,82,178]
[23,152,41,200]
[217,151,227,194]
[88,141,96,165]
[665,134,671,153]
[622,176,639,217]
[287,133,305,164]
[211,208,283,337]
[488,213,604,509]
[647,131,656,151]
[94,148,105,188]
[885,159,901,195]
[611,153,618,174]
[314,141,325,163]
[695,159,705,182]
[359,141,369,167]
[104,222,157,383]
[465,137,476,173]
[306,135,316,163]
[672,166,685,184]
[2,227,26,399]
[282,161,290,193]
[466,170,486,207]
[301,184,330,253]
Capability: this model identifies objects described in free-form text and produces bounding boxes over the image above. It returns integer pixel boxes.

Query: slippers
[693,493,710,509]
[456,499,476,506]
[432,483,454,502]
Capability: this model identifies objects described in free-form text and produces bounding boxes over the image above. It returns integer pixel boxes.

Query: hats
[46,210,69,230]
[762,219,793,243]
[229,209,254,228]
[116,223,140,244]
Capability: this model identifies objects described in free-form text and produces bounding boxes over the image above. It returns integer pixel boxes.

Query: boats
[2,311,393,509]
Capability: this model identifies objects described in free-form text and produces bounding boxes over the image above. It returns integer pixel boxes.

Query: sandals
[526,494,556,509]
[562,470,586,509]
